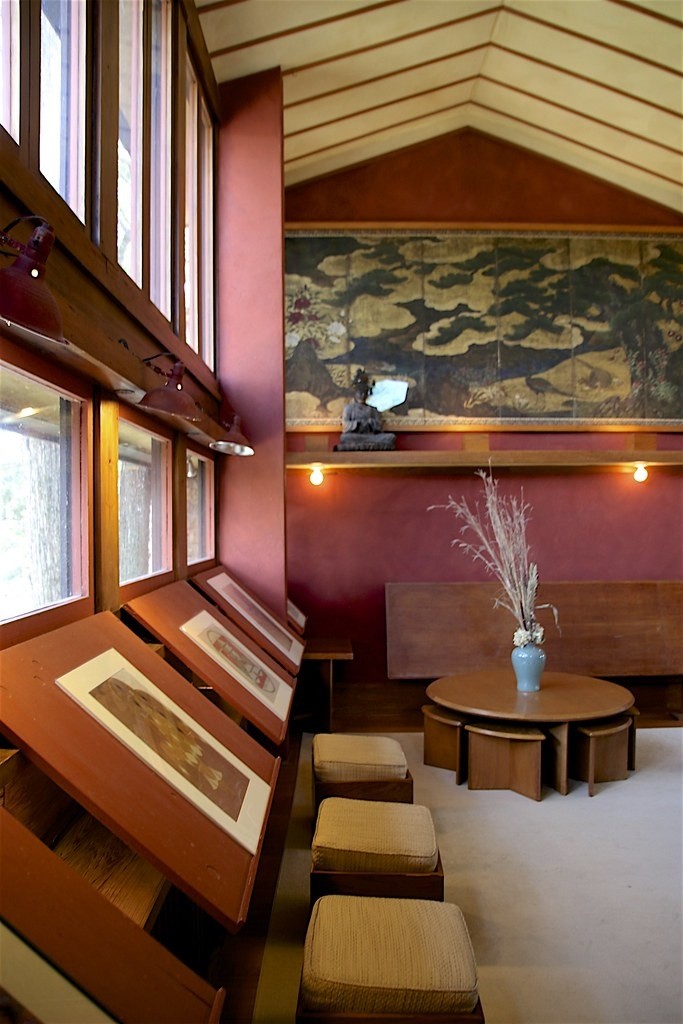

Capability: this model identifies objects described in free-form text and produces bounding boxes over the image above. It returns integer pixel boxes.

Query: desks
[430,667,635,793]
[296,635,354,733]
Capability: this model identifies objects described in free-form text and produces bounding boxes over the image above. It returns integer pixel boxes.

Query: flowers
[427,460,561,647]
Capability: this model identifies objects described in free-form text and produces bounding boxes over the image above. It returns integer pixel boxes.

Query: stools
[310,733,415,816]
[295,897,487,1023]
[310,796,447,913]
[421,705,641,802]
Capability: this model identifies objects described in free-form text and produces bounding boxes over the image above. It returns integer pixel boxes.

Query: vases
[513,641,547,692]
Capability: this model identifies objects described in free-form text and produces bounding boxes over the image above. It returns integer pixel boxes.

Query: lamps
[0,216,67,344]
[209,412,253,456]
[141,352,201,423]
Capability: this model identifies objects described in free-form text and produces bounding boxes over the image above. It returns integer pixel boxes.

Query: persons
[338,369,397,450]
